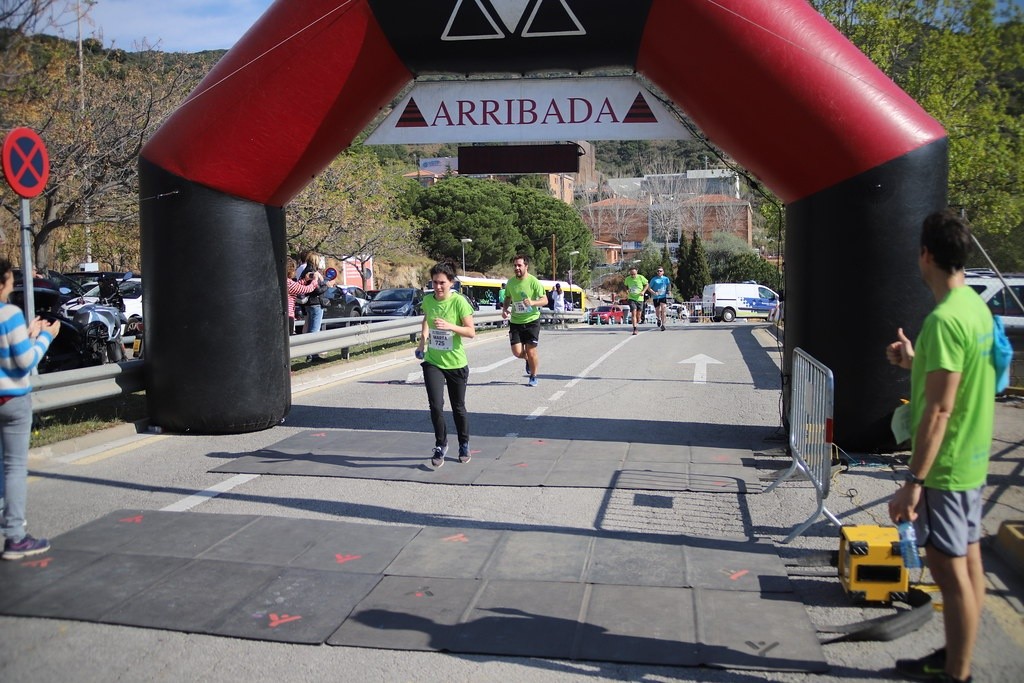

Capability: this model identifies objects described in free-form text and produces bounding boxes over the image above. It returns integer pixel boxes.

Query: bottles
[898,517,921,567]
[523,299,532,313]
[415,351,424,359]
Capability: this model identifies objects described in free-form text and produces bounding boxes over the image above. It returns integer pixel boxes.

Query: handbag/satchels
[320,297,331,308]
[992,313,1013,393]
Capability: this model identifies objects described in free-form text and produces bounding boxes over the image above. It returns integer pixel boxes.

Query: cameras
[309,274,314,278]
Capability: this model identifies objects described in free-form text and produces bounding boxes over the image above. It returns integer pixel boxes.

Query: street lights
[569,251,579,311]
[461,238,472,276]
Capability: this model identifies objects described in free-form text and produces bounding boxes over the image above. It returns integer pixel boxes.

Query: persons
[648,266,670,330]
[415,263,475,467]
[888,209,996,683]
[690,293,700,319]
[548,283,564,324]
[499,283,506,307]
[287,251,329,363]
[624,267,649,334]
[0,258,59,560]
[502,256,547,386]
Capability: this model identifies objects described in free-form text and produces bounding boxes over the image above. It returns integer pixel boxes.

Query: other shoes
[307,356,311,361]
[312,357,328,364]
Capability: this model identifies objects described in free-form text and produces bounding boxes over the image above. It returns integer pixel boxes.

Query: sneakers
[661,325,665,331]
[526,360,531,374]
[2,533,50,560]
[458,441,471,464]
[895,648,973,683]
[431,443,449,467]
[641,314,644,320]
[633,328,638,334]
[657,319,661,327]
[528,375,538,387]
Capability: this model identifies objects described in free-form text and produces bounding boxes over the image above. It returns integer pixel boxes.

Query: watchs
[906,471,925,485]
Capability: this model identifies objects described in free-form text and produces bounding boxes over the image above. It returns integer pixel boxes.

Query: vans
[703,283,782,323]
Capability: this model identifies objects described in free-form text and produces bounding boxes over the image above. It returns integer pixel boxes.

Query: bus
[455,275,584,326]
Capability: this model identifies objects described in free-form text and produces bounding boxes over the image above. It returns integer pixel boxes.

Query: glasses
[657,270,662,272]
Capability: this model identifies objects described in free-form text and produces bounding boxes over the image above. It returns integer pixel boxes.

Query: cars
[963,272,1024,328]
[627,305,661,325]
[7,267,428,373]
[573,307,593,323]
[589,305,624,324]
[596,261,625,272]
[666,305,689,319]
